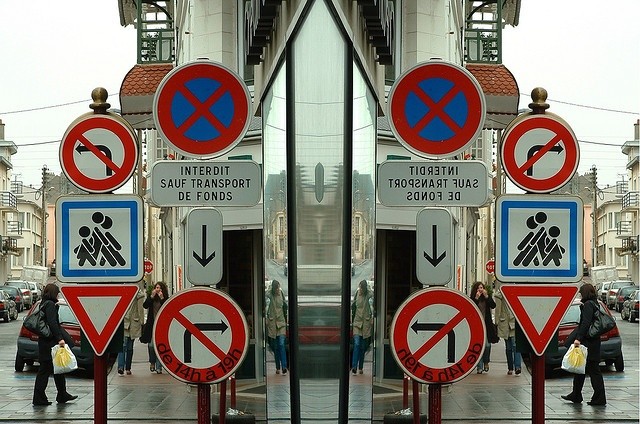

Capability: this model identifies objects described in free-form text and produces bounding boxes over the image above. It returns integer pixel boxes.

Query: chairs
[283,368,287,373]
[352,369,356,374]
[516,368,521,374]
[126,369,131,375]
[118,369,124,374]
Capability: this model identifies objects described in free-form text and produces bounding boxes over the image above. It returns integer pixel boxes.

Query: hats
[0,290,19,323]
[620,291,639,323]
[25,280,44,304]
[596,280,632,303]
[519,299,624,378]
[606,282,636,309]
[615,287,639,312]
[3,281,33,310]
[15,298,111,374]
[0,286,25,313]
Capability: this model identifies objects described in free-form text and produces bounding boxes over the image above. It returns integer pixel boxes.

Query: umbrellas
[587,400,593,405]
[45,402,52,405]
[68,396,78,400]
[485,363,489,372]
[561,394,570,400]
[150,363,155,371]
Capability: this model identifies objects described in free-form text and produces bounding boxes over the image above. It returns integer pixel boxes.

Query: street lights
[281,291,287,323]
[139,322,152,343]
[352,291,358,321]
[586,300,617,339]
[560,344,588,375]
[23,300,51,338]
[487,322,499,343]
[51,343,78,374]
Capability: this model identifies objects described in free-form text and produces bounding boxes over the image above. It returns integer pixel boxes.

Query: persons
[117,293,144,374]
[265,279,287,375]
[33,283,78,405]
[470,281,496,373]
[352,279,375,375]
[561,283,606,405]
[494,291,521,376]
[140,281,170,373]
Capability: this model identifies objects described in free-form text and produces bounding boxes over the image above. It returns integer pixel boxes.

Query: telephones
[379,160,488,208]
[150,159,262,207]
